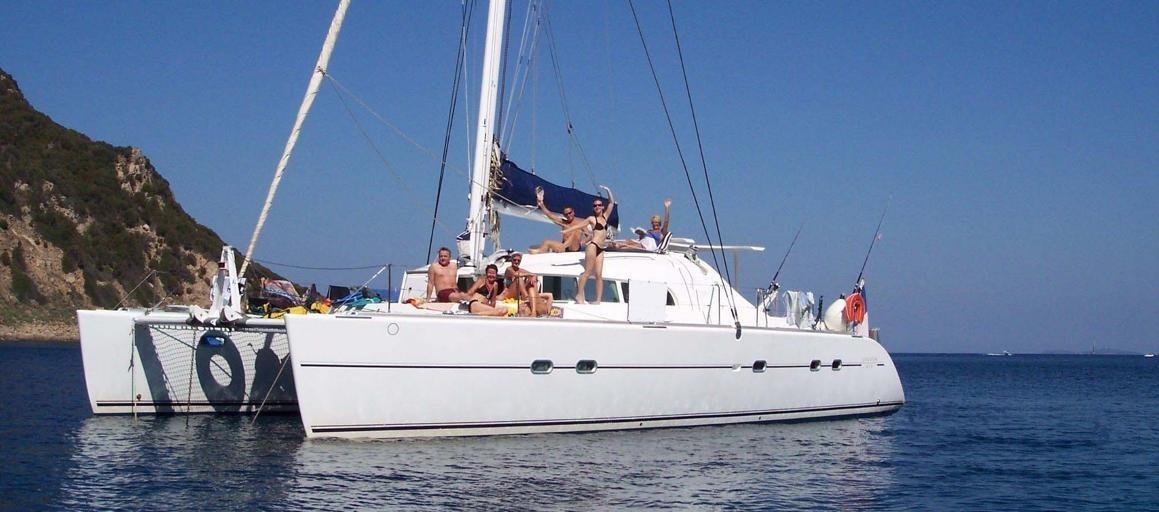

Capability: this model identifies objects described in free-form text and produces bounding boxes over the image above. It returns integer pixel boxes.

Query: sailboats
[74,0,905,442]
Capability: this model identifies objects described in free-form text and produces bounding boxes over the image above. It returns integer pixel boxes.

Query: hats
[510,252,524,257]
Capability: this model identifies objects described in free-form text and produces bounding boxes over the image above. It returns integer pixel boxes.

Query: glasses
[513,259,521,262]
[593,204,602,207]
[565,211,574,216]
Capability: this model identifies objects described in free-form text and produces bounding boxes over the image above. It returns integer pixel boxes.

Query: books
[630,227,647,235]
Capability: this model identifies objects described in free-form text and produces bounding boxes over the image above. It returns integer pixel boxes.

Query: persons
[527,186,593,256]
[558,184,615,307]
[517,285,555,318]
[605,198,671,252]
[300,283,333,314]
[365,291,382,303]
[248,299,273,319]
[503,250,539,299]
[465,263,509,317]
[422,247,509,304]
